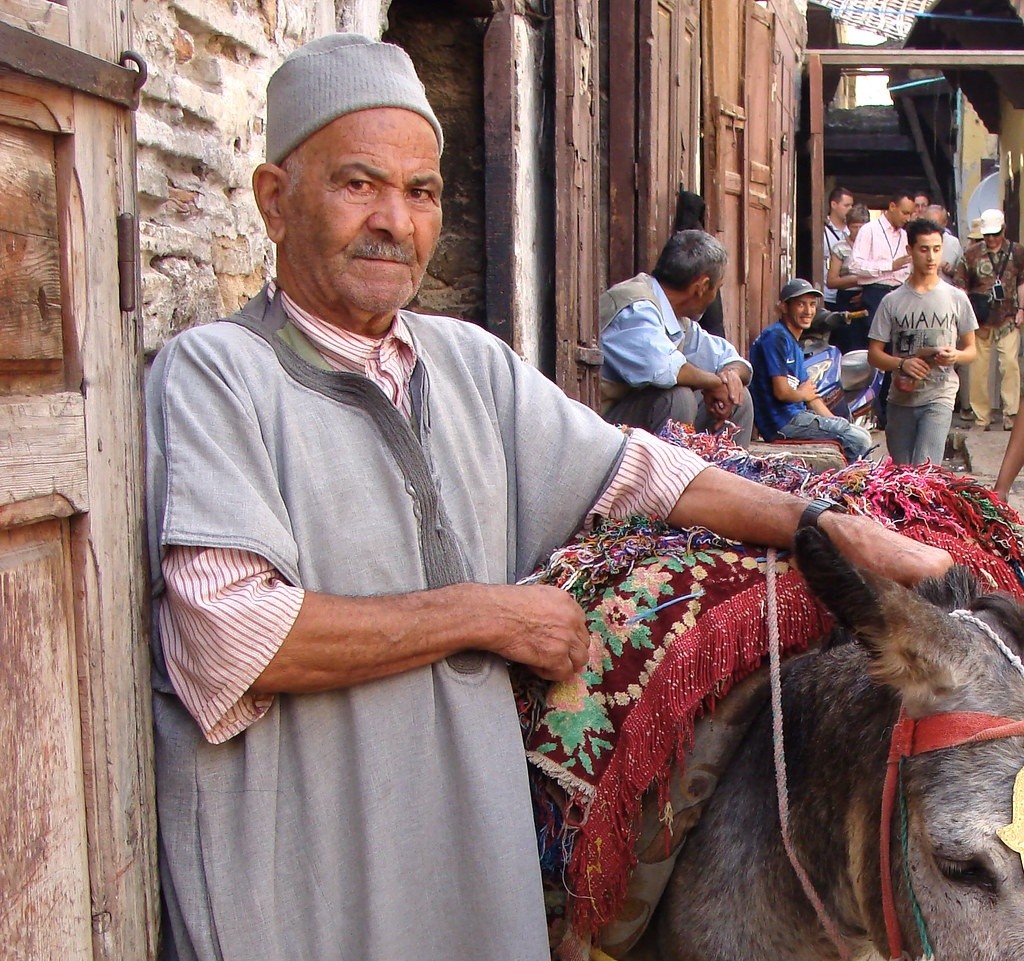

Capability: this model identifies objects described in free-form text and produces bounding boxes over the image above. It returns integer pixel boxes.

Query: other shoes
[959,408,974,420]
[970,424,991,432]
[1001,415,1015,431]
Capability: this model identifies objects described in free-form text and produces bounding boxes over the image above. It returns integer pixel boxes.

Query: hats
[968,217,983,239]
[264,32,443,164]
[980,209,1004,235]
[779,278,823,302]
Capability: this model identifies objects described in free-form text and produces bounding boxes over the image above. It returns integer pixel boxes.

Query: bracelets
[1019,308,1024,310]
[898,359,905,375]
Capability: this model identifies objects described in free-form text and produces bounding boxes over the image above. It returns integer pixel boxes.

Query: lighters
[718,401,724,409]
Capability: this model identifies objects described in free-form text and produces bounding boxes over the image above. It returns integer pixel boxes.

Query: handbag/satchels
[967,292,992,324]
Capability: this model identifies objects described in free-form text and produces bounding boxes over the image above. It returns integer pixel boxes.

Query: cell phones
[994,285,1004,300]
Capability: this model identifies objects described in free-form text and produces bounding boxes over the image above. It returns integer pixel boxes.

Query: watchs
[797,497,847,532]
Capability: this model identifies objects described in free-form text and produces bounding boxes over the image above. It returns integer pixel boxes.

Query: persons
[749,278,873,466]
[600,229,755,449]
[142,32,953,961]
[867,219,980,467]
[821,186,963,430]
[992,396,1024,503]
[953,208,1024,431]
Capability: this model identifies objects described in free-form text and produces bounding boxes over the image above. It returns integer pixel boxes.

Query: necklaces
[879,219,902,259]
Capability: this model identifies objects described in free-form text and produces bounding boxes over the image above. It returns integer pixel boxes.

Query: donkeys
[630,521,1024,961]
